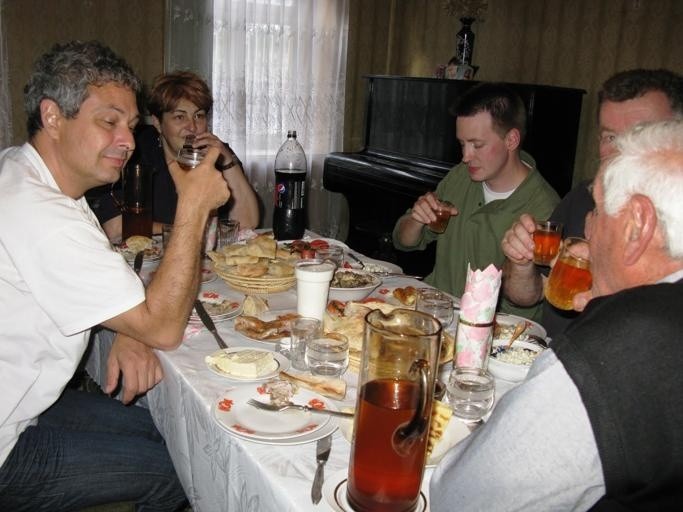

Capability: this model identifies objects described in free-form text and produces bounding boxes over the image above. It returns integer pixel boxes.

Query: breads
[394,285,418,306]
[426,403,450,459]
[126,236,154,253]
[201,232,299,283]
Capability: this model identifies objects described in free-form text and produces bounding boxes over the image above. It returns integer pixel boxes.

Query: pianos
[323,75,587,279]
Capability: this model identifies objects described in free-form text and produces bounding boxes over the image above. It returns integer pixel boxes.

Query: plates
[424,420,460,468]
[184,286,245,325]
[333,323,454,367]
[273,238,349,256]
[349,260,401,275]
[321,468,427,512]
[206,308,340,445]
[147,268,218,284]
[491,312,547,343]
[125,247,165,263]
[488,339,541,383]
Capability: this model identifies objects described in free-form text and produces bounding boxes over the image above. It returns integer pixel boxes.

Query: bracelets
[219,159,239,170]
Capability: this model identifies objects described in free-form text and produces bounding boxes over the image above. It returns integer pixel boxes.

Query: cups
[173,133,209,173]
[294,261,334,320]
[428,199,453,233]
[344,308,442,512]
[434,378,446,402]
[414,287,452,327]
[161,224,172,248]
[315,244,344,268]
[531,219,561,266]
[447,369,493,419]
[543,237,595,311]
[108,160,154,243]
[218,219,240,249]
[304,333,351,378]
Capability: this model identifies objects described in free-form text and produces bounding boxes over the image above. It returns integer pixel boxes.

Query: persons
[428,117,682,511]
[0,41,229,511]
[95,67,259,244]
[389,83,560,328]
[495,69,683,339]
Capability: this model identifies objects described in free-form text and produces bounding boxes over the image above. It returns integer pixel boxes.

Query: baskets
[213,260,297,296]
[348,330,455,374]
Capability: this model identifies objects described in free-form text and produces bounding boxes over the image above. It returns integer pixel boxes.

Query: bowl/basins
[328,270,382,304]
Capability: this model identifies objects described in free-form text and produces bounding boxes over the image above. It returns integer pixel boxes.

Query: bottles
[272,131,307,239]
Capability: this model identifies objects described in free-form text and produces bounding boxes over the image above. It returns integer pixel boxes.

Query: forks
[246,398,354,420]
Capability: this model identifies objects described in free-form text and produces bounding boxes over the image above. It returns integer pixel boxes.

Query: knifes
[133,249,144,275]
[346,252,364,269]
[310,435,332,505]
[192,300,227,349]
[375,272,424,278]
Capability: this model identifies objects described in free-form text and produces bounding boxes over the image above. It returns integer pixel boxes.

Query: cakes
[214,293,454,400]
